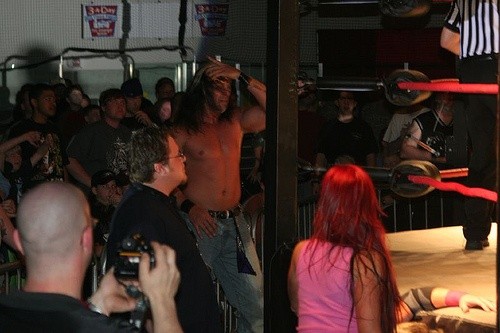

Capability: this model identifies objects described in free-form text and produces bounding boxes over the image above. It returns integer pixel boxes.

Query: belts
[208,207,240,220]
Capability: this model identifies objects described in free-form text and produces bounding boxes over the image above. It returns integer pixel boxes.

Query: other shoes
[466,238,489,250]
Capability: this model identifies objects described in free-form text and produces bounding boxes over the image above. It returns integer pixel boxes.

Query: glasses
[45,97,57,101]
[167,152,184,160]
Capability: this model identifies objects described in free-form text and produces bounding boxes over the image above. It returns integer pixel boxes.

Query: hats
[121,79,143,97]
[91,169,116,184]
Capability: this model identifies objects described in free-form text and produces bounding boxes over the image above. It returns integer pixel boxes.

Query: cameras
[115,234,157,280]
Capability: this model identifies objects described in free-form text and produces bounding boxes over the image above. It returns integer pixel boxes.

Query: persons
[378,0,433,77]
[0,76,176,293]
[106,127,230,333]
[395,286,497,333]
[0,180,184,333]
[289,162,410,333]
[267,71,383,265]
[440,0,500,250]
[357,92,472,234]
[168,56,266,333]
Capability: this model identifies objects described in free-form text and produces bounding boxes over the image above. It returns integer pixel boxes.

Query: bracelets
[238,73,253,87]
[179,199,196,214]
[86,299,109,317]
[445,290,468,306]
[38,150,44,156]
